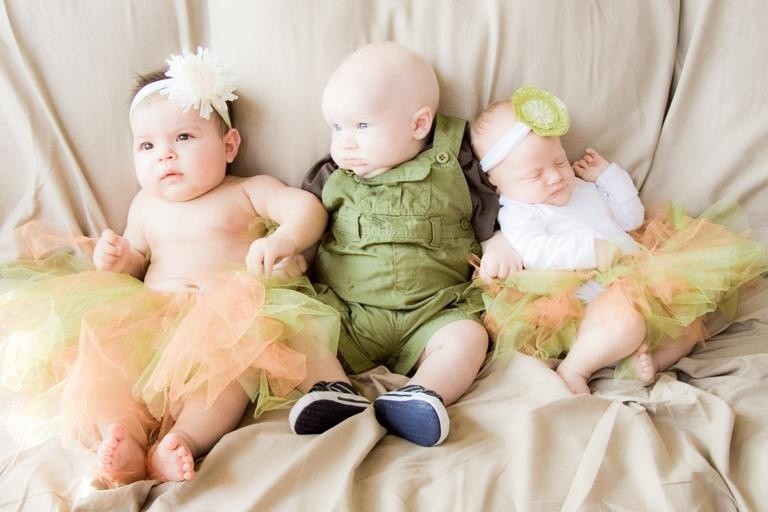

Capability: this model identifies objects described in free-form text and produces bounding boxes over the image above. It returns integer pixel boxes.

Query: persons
[266,42,523,447]
[465,98,710,395]
[90,62,329,485]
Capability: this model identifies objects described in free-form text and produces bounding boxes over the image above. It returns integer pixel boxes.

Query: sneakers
[374,385,450,447]
[288,381,371,434]
